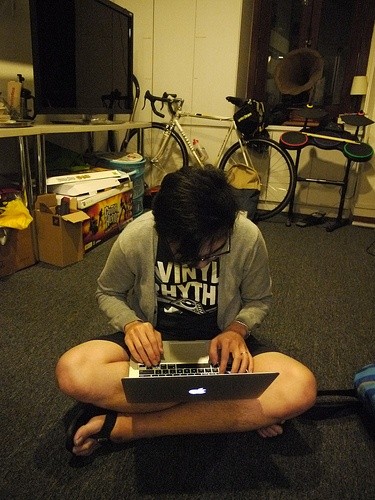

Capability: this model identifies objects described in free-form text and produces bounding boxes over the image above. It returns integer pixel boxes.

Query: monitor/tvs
[30,0,134,123]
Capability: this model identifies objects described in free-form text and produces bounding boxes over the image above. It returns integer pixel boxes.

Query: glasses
[164,231,232,269]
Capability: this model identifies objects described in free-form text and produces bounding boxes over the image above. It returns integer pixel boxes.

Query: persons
[56,163,317,457]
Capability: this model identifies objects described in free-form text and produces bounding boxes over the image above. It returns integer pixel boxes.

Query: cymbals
[340,114,374,126]
[292,106,329,119]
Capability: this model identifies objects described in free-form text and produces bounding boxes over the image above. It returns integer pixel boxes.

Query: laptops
[121,340,279,403]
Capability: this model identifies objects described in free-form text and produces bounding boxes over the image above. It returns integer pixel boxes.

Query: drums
[311,130,343,150]
[280,131,309,150]
[343,141,374,163]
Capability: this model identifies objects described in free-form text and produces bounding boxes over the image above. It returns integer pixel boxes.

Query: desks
[0,118,152,262]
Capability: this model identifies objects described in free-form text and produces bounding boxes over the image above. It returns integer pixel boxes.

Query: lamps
[350,76,368,112]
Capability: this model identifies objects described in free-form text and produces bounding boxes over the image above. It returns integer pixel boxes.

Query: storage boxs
[53,167,133,209]
[0,222,36,279]
[35,194,91,268]
[82,181,136,253]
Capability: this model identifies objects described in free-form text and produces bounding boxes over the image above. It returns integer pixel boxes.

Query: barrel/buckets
[96,152,147,218]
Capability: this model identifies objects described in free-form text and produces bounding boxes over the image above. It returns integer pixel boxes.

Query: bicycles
[119,90,297,221]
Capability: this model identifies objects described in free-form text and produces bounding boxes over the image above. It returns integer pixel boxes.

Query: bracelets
[233,319,250,340]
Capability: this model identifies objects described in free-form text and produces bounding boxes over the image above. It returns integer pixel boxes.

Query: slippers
[64,405,129,458]
